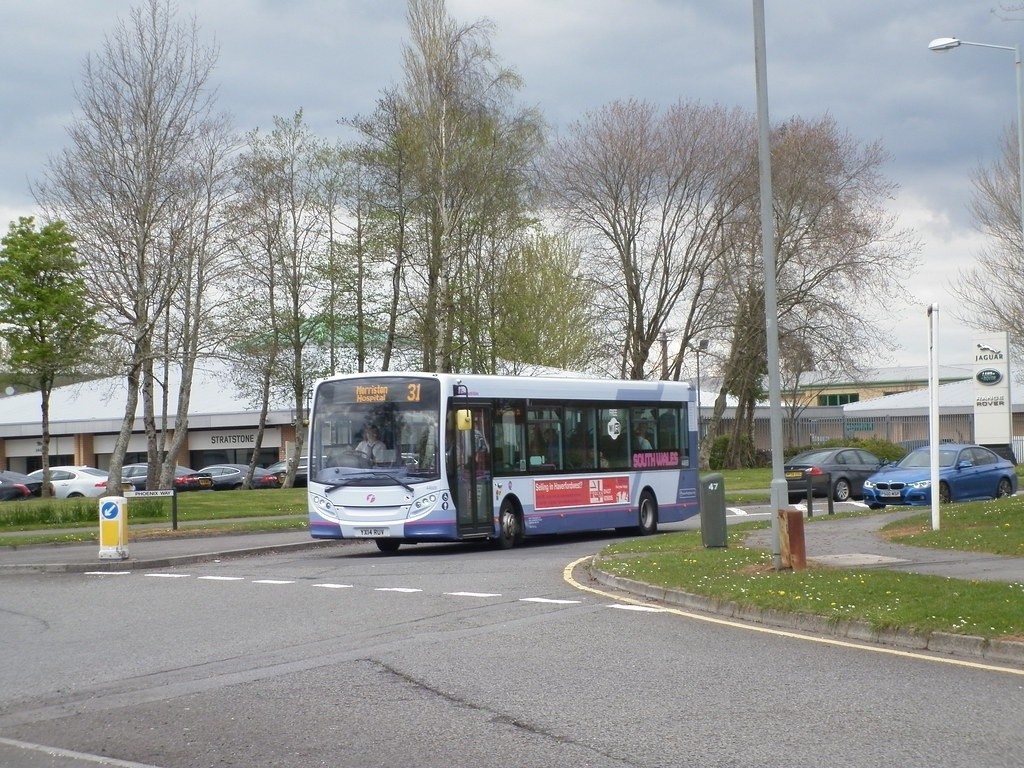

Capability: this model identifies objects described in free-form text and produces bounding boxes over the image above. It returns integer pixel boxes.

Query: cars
[863,443,1019,512]
[785,446,891,501]
[121,461,214,493]
[268,455,326,482]
[401,454,420,466]
[26,465,136,499]
[0,470,55,504]
[198,463,279,491]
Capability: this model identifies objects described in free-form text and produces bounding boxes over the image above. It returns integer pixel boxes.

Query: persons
[355,425,387,462]
[581,445,609,469]
[544,427,565,466]
[636,428,652,449]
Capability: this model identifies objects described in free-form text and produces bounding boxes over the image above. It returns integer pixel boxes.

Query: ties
[368,444,375,459]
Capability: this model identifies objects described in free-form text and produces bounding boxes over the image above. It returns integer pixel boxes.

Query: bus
[300,374,701,553]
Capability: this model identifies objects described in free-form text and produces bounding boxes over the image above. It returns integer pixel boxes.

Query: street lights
[687,340,711,427]
[929,37,1023,214]
